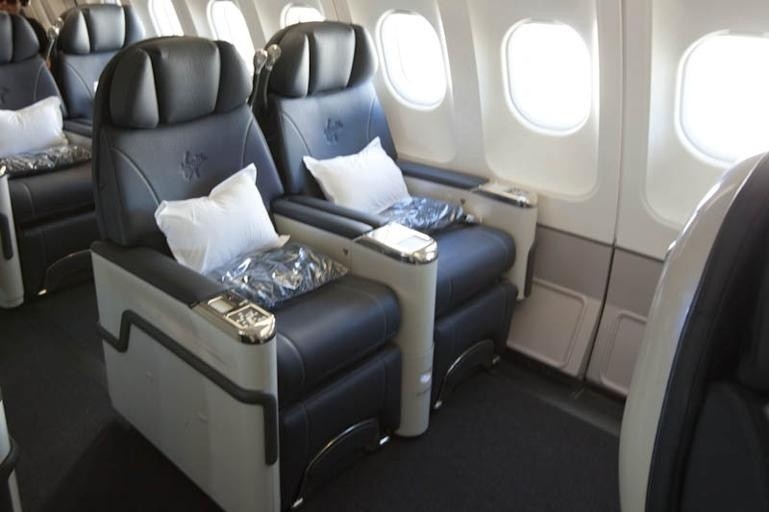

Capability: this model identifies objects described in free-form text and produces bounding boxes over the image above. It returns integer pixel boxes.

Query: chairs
[50,4,150,127]
[248,19,540,438]
[89,33,440,512]
[1,10,95,314]
[618,151,769,512]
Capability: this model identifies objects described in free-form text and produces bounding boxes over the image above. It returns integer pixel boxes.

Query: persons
[0,0,53,71]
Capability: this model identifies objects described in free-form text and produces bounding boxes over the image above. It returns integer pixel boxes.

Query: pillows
[0,96,69,159]
[153,161,291,277]
[302,136,413,215]
